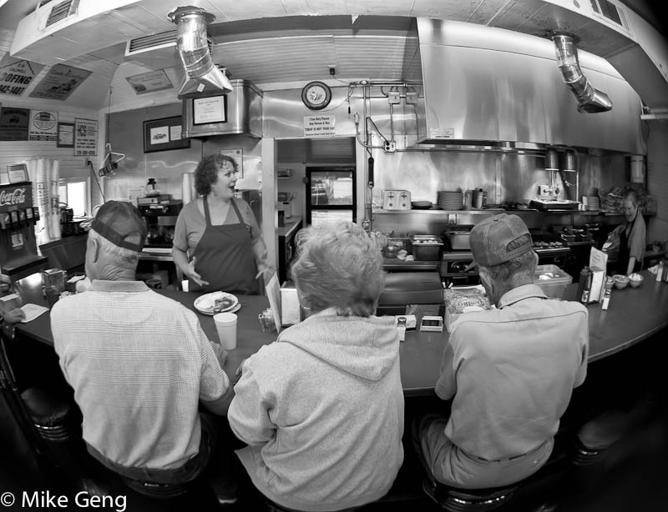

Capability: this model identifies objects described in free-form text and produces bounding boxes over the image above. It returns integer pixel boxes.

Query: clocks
[301,80,331,112]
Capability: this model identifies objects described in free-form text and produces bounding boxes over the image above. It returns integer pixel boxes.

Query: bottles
[655,261,663,282]
[600,292,610,311]
[398,318,406,342]
[606,288,611,300]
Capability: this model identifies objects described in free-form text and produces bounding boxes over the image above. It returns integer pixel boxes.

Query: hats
[464,212,535,274]
[89,200,148,253]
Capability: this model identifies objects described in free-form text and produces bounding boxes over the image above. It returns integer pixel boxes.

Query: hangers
[101,142,126,171]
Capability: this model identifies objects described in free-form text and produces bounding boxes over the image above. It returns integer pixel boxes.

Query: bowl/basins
[628,273,645,288]
[612,274,630,289]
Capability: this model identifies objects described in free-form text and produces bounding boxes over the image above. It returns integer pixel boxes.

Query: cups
[214,312,238,350]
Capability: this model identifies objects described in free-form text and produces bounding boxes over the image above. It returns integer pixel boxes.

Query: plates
[436,191,464,212]
[194,291,242,315]
[583,195,600,212]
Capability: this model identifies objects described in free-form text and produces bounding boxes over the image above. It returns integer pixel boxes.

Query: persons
[615,191,644,272]
[170,156,275,295]
[416,213,586,489]
[50,200,234,499]
[228,221,406,512]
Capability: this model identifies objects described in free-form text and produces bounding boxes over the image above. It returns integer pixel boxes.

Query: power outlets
[384,141,396,153]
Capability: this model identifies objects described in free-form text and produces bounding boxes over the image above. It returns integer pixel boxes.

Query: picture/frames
[191,94,228,125]
[141,115,191,153]
[56,122,76,148]
